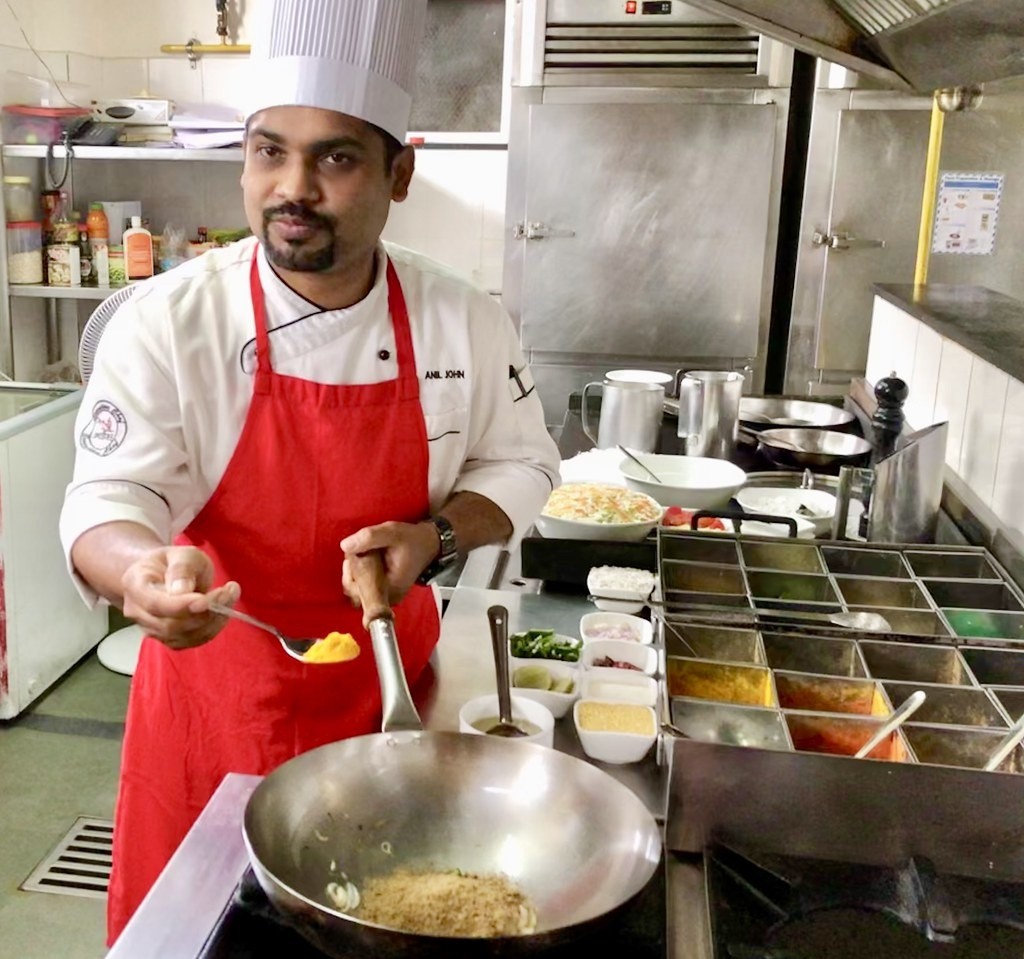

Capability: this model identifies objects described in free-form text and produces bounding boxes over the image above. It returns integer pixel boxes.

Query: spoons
[153,583,360,663]
[740,410,813,425]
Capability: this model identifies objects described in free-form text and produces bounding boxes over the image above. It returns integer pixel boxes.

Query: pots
[244,549,663,959]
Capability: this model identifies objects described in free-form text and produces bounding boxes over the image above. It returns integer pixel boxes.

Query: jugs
[678,371,745,465]
[582,370,673,452]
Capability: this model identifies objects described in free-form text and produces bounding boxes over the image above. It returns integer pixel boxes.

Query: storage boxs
[0,99,180,144]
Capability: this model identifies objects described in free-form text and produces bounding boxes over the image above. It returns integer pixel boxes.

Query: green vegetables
[511,630,581,661]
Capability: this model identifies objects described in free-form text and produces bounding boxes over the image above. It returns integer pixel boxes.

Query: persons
[59,0,560,946]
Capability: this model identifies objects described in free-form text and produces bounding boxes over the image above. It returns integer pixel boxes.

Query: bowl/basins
[535,484,662,542]
[739,398,855,432]
[659,507,735,533]
[574,700,657,763]
[581,671,658,706]
[581,612,653,644]
[619,455,746,512]
[737,487,837,536]
[510,663,580,718]
[582,640,658,677]
[757,428,872,477]
[508,632,582,668]
[587,568,654,614]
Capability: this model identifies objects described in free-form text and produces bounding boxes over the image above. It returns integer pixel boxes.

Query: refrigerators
[0,382,109,719]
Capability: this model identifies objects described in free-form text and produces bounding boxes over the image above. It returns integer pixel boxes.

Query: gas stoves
[192,841,1024,959]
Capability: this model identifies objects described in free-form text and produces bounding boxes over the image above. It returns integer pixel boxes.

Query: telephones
[58,115,125,146]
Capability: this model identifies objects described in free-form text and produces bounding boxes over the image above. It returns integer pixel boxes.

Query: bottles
[3,177,35,222]
[197,228,207,243]
[53,192,77,247]
[86,203,109,259]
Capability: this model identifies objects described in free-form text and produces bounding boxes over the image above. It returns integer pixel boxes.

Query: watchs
[418,514,462,584]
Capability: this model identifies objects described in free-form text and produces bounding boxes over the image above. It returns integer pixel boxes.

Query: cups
[6,222,44,283]
[459,694,554,749]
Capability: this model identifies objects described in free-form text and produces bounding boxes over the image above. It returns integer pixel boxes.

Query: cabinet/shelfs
[0,144,245,383]
